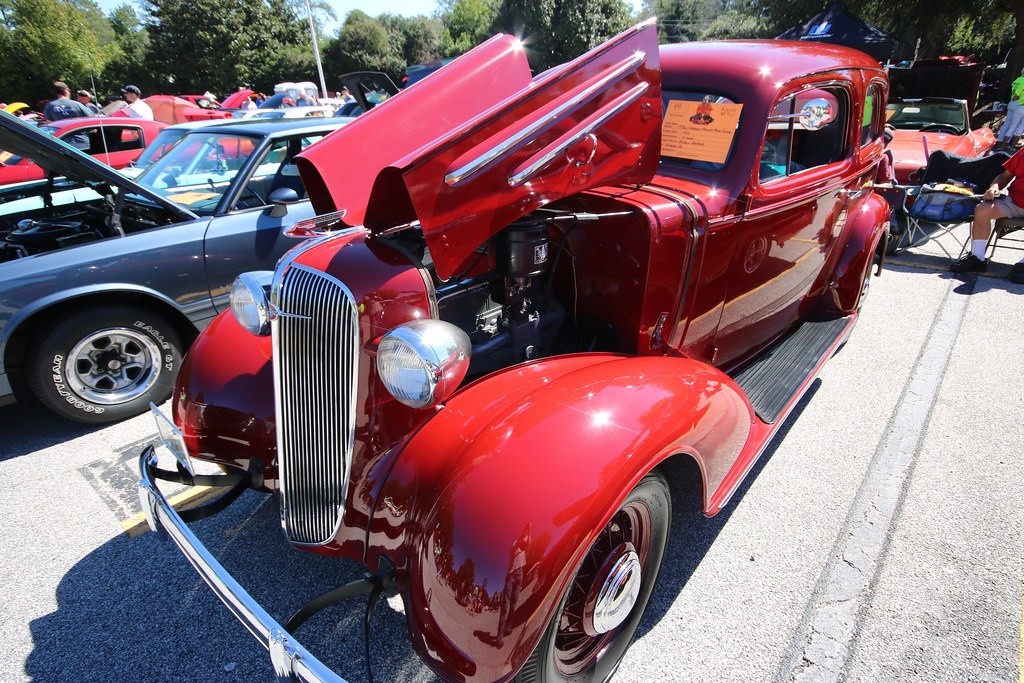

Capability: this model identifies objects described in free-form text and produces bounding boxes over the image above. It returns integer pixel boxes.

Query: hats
[121,85,141,97]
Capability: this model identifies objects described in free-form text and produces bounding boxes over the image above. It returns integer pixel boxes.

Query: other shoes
[995,147,1018,152]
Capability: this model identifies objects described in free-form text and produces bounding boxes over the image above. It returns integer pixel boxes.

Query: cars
[0,105,363,427]
[0,117,259,185]
[139,17,898,683]
[770,85,875,123]
[866,49,1010,207]
[141,58,457,126]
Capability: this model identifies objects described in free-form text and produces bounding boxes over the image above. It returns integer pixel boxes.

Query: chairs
[985,219,1024,262]
[891,150,1011,264]
[268,153,306,201]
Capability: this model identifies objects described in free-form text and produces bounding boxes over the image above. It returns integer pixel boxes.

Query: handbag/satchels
[911,182,974,221]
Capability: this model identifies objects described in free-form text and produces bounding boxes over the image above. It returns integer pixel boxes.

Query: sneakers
[950,251,987,273]
[1009,263,1024,284]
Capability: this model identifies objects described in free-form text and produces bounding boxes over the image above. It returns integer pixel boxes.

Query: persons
[0,82,154,156]
[207,86,356,110]
[877,76,1024,284]
